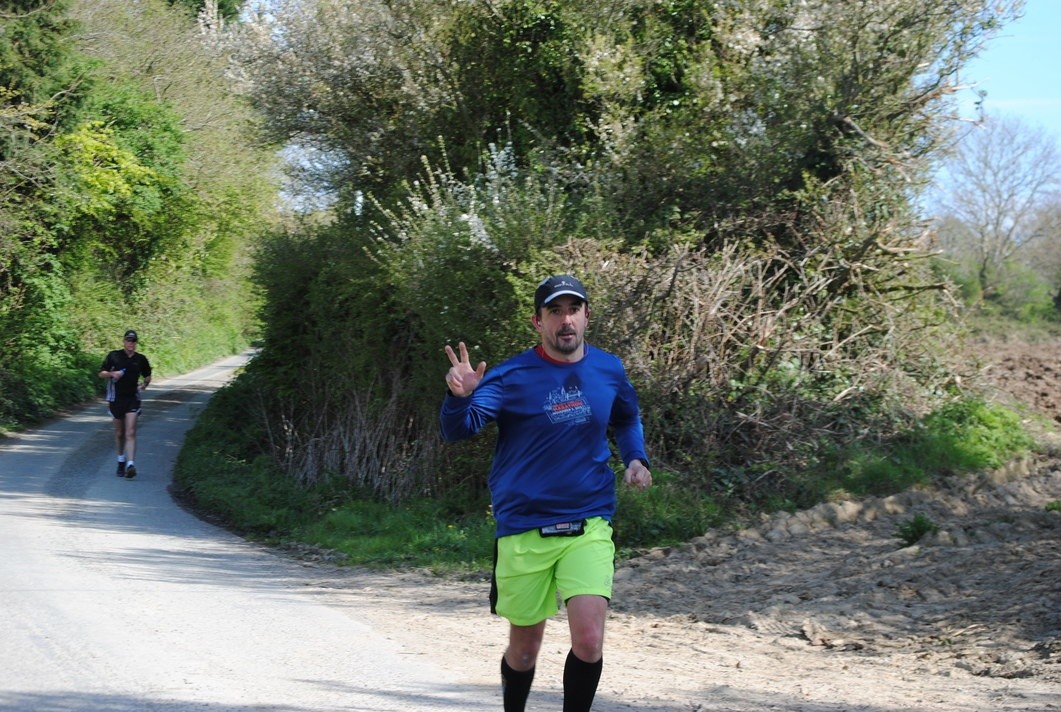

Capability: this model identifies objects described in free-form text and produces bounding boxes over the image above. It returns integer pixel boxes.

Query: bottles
[112,368,126,382]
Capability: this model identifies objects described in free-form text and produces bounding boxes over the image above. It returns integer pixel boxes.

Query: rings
[642,483,645,488]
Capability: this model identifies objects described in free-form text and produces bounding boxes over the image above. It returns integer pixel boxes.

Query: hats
[125,330,137,341]
[533,275,589,311]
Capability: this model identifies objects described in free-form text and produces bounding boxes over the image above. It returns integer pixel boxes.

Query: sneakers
[117,462,126,477]
[125,465,137,479]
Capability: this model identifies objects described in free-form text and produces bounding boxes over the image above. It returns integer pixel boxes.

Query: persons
[97,331,153,478]
[437,274,654,712]
